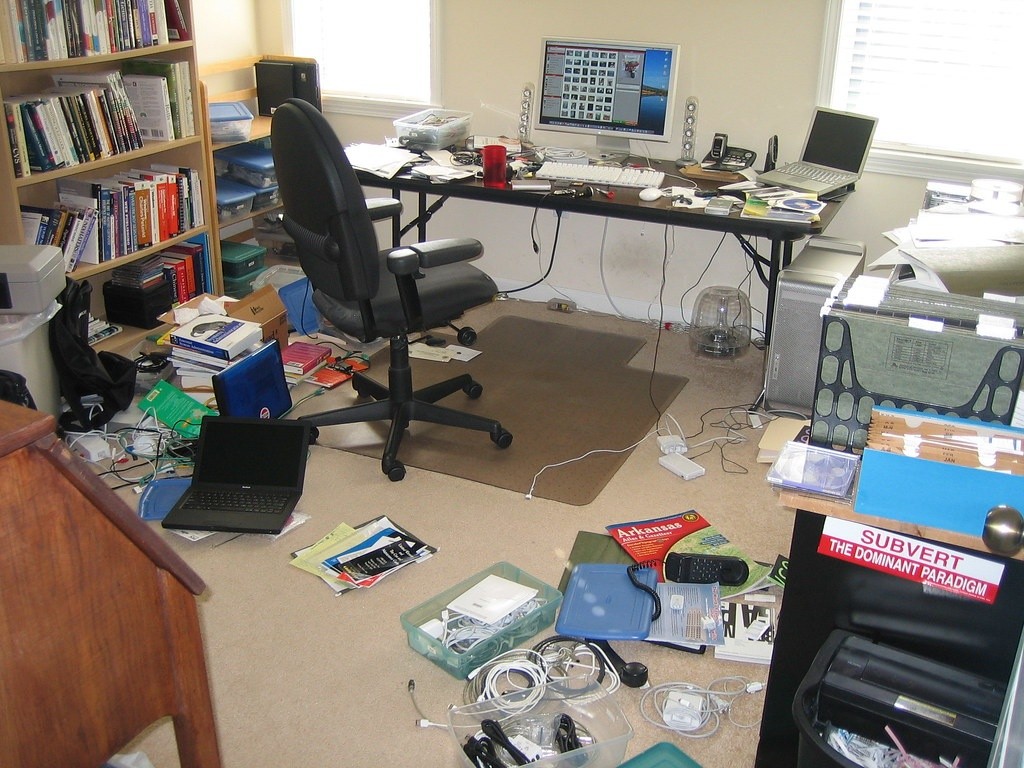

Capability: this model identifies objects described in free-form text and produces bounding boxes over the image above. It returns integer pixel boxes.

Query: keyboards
[536,161,665,188]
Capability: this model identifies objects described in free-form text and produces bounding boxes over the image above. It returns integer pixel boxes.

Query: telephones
[702,132,757,173]
[585,638,648,688]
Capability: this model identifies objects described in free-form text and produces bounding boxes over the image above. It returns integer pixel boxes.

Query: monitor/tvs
[533,36,681,164]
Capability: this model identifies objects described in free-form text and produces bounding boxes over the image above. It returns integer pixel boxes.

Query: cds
[783,199,821,210]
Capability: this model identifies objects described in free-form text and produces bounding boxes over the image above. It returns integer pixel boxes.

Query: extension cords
[67,434,111,463]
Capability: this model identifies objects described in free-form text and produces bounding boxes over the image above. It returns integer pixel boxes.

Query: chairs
[272,97,513,482]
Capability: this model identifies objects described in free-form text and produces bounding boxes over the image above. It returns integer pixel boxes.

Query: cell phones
[659,452,705,480]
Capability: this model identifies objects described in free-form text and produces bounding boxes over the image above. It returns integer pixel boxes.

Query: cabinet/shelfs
[1,0,218,356]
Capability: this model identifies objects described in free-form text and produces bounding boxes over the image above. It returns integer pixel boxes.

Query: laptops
[161,416,313,535]
[756,105,879,197]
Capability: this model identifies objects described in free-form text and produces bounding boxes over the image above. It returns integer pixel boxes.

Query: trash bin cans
[0,298,63,425]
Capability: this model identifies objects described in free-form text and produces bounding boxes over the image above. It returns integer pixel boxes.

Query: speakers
[517,83,535,142]
[675,95,699,167]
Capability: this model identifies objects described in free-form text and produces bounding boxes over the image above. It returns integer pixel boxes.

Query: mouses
[639,187,663,202]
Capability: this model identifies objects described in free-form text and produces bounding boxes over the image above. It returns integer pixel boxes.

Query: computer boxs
[762,235,866,420]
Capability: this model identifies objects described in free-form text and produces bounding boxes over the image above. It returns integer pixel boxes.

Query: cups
[484,145,507,189]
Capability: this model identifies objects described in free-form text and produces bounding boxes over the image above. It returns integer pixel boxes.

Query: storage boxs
[102,279,174,330]
[400,561,563,680]
[156,264,324,349]
[204,102,255,144]
[0,245,67,315]
[215,176,258,224]
[212,144,278,187]
[448,672,634,768]
[222,169,280,209]
[219,240,268,277]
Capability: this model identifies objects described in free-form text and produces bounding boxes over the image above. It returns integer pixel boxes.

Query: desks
[754,416,1024,766]
[350,138,846,413]
[198,53,320,295]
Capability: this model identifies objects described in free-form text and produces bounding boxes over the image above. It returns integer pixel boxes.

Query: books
[756,415,811,463]
[289,515,437,592]
[111,232,213,305]
[87,313,122,346]
[605,510,790,664]
[740,192,820,224]
[138,379,217,439]
[146,313,367,388]
[4,60,195,178]
[473,135,521,153]
[20,163,205,272]
[0,0,190,64]
